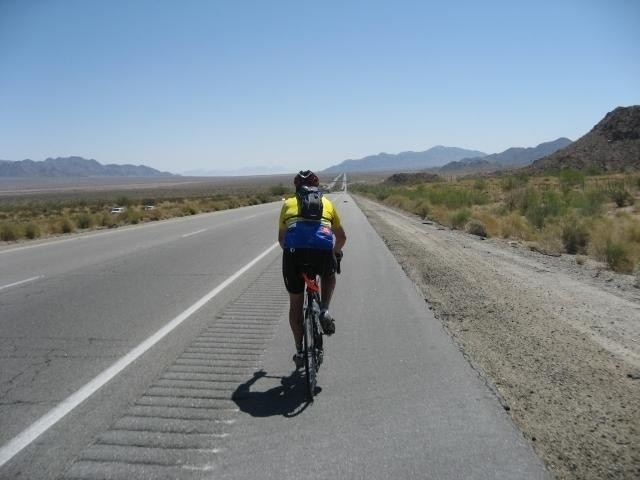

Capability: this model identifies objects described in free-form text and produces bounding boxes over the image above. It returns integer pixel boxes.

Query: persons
[278,170,346,360]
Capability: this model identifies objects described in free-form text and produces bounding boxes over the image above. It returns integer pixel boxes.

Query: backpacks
[296,185,323,220]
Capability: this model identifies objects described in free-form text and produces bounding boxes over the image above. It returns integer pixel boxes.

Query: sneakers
[318,311,335,334]
[293,354,305,367]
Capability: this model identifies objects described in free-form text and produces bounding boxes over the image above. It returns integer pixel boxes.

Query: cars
[110,207,124,214]
[320,184,332,193]
[146,206,155,211]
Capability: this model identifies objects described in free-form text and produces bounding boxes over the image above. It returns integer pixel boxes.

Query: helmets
[294,170,319,186]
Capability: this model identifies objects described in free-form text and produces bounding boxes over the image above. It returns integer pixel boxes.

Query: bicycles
[293,249,343,403]
